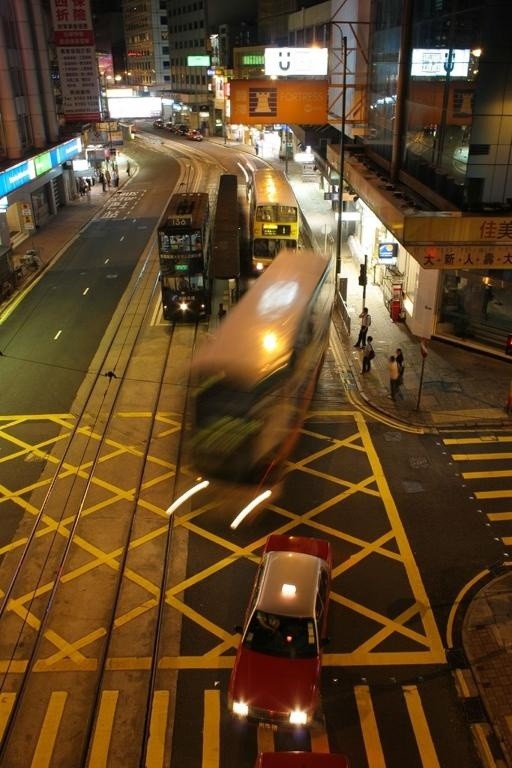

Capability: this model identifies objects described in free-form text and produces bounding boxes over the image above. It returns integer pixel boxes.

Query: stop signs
[419,339,428,358]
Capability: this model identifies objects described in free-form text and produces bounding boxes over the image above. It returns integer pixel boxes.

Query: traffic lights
[358,261,367,286]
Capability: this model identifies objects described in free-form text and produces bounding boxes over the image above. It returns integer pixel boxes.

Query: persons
[224,131,228,144]
[71,157,131,197]
[254,142,259,155]
[352,307,371,349]
[254,210,295,261]
[387,354,401,401]
[396,348,404,378]
[359,336,374,375]
[216,303,227,321]
[257,609,282,634]
[163,230,203,297]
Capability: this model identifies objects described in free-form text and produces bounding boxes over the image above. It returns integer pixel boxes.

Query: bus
[160,191,212,323]
[171,247,333,529]
[246,163,301,275]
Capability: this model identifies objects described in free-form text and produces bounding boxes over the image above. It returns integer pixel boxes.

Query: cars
[227,533,332,730]
[152,118,204,141]
[259,750,351,768]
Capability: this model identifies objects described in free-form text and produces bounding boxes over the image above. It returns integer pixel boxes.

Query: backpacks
[367,345,375,360]
